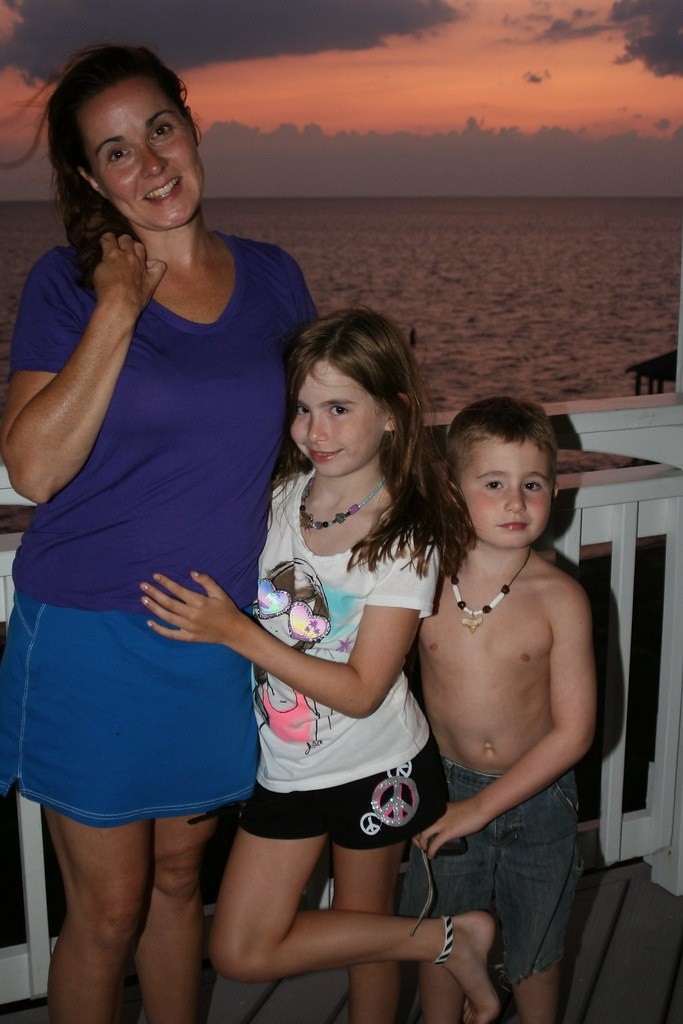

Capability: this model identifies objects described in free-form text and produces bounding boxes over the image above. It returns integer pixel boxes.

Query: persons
[140,308,500,1024]
[0,42,319,1024]
[399,397,598,1023]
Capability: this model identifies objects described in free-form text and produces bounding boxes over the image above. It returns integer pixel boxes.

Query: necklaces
[451,547,531,634]
[300,471,385,529]
